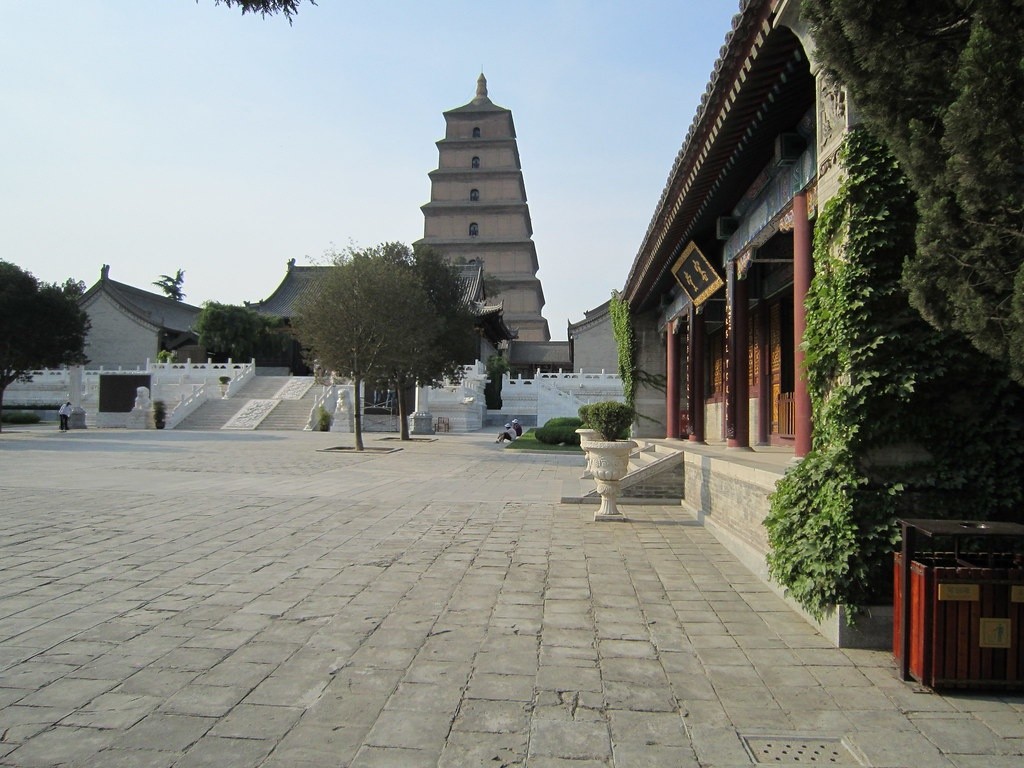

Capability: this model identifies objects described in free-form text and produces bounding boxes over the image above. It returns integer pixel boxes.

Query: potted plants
[580,401,639,522]
[218,376,231,397]
[153,401,167,429]
[575,404,595,479]
[318,407,331,432]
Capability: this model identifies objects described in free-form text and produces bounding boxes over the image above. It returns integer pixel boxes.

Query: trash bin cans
[892,517,1024,694]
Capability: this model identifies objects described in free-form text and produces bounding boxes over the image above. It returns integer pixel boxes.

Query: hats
[504,423,511,427]
[512,419,518,423]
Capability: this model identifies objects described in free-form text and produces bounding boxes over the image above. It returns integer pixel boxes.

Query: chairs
[435,417,449,432]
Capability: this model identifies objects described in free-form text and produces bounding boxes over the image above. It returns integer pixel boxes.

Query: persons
[496,423,517,443]
[512,418,522,436]
[58,402,72,430]
